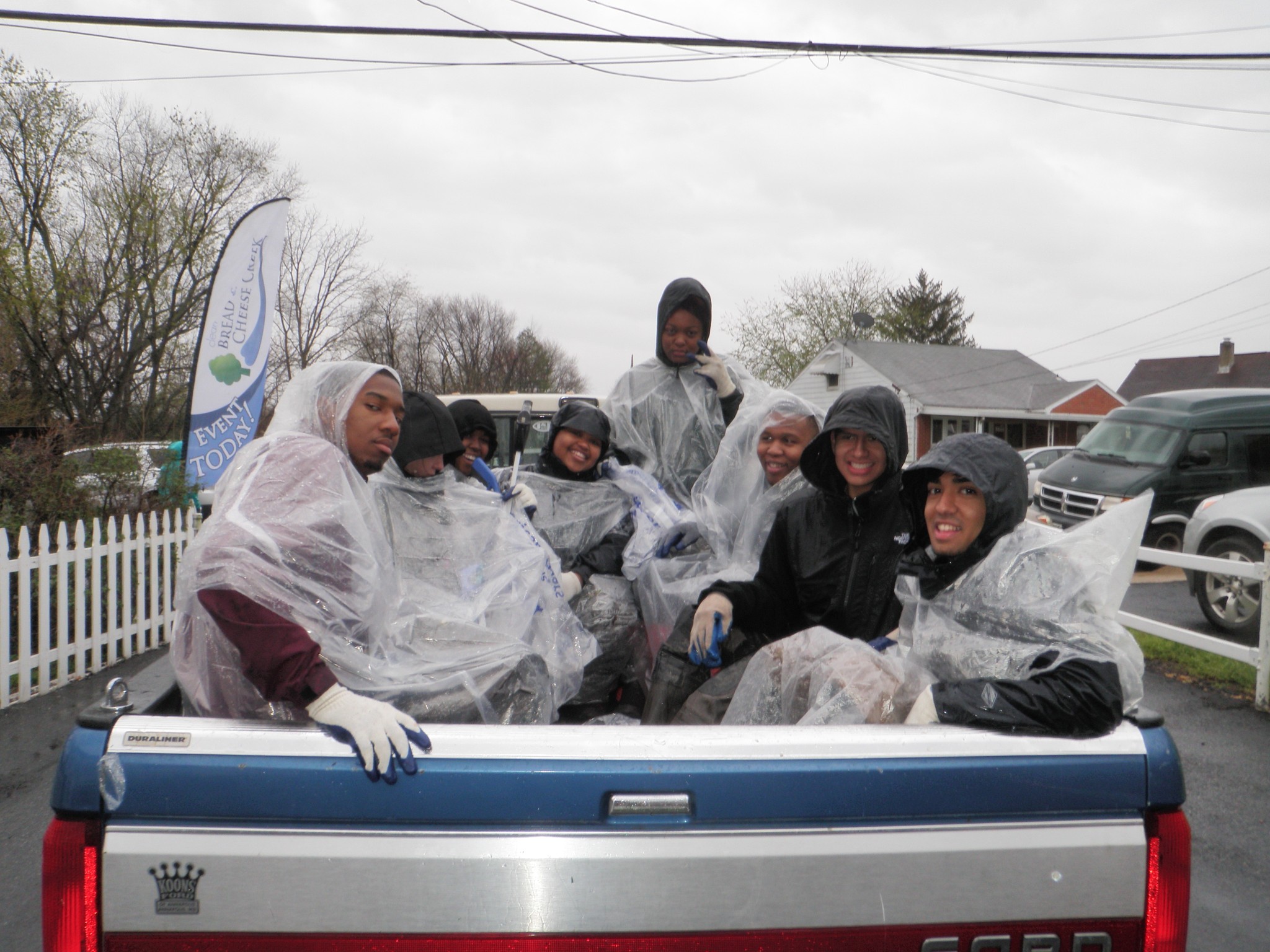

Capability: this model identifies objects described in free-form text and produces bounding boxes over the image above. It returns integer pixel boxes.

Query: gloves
[653,521,709,559]
[560,571,582,602]
[685,338,737,400]
[687,591,734,669]
[501,483,538,522]
[305,681,431,785]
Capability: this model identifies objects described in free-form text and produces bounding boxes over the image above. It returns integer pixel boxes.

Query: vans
[1031,387,1270,573]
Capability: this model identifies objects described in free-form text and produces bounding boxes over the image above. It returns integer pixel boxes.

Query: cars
[1017,445,1077,504]
[1180,486,1269,645]
[62,441,174,521]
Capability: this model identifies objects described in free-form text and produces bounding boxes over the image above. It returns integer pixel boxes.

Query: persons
[601,277,756,515]
[170,360,1156,785]
